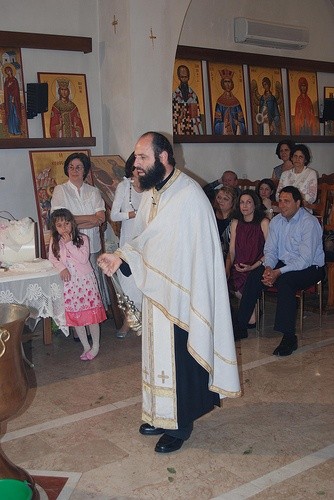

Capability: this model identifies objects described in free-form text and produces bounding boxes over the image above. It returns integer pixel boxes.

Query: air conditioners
[233,16,312,49]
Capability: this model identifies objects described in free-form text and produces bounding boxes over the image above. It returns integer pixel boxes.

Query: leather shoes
[273,334,297,356]
[139,423,164,434]
[155,432,190,453]
[233,327,248,339]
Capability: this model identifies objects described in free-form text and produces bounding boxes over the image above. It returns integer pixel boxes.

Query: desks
[0,258,70,344]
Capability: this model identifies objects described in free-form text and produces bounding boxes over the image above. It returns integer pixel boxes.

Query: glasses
[67,164,85,172]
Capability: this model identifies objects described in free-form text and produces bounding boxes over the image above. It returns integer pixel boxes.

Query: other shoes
[116,329,128,338]
[323,305,334,311]
[80,351,95,361]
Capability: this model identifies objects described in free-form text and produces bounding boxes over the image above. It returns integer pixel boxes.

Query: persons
[90,161,122,239]
[48,79,85,138]
[48,208,108,361]
[202,141,334,356]
[213,68,246,136]
[108,150,143,338]
[108,159,127,182]
[1,61,22,135]
[47,152,110,342]
[95,131,244,454]
[255,77,282,137]
[171,65,204,135]
[293,77,318,137]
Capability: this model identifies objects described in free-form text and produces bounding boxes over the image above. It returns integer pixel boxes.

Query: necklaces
[292,167,308,174]
[129,181,138,214]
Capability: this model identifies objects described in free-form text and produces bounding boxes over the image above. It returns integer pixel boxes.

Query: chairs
[202,173,334,335]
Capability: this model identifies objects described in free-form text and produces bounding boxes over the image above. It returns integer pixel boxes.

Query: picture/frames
[0,46,29,138]
[28,148,127,256]
[248,67,284,135]
[323,87,334,136]
[203,62,247,136]
[172,61,205,135]
[286,69,321,135]
[37,72,92,140]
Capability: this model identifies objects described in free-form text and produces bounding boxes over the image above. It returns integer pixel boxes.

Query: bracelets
[259,259,263,263]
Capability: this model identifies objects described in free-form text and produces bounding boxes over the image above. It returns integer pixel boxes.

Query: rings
[64,278,70,281]
[63,235,70,239]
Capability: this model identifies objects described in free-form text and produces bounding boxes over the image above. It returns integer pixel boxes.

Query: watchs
[266,208,274,214]
